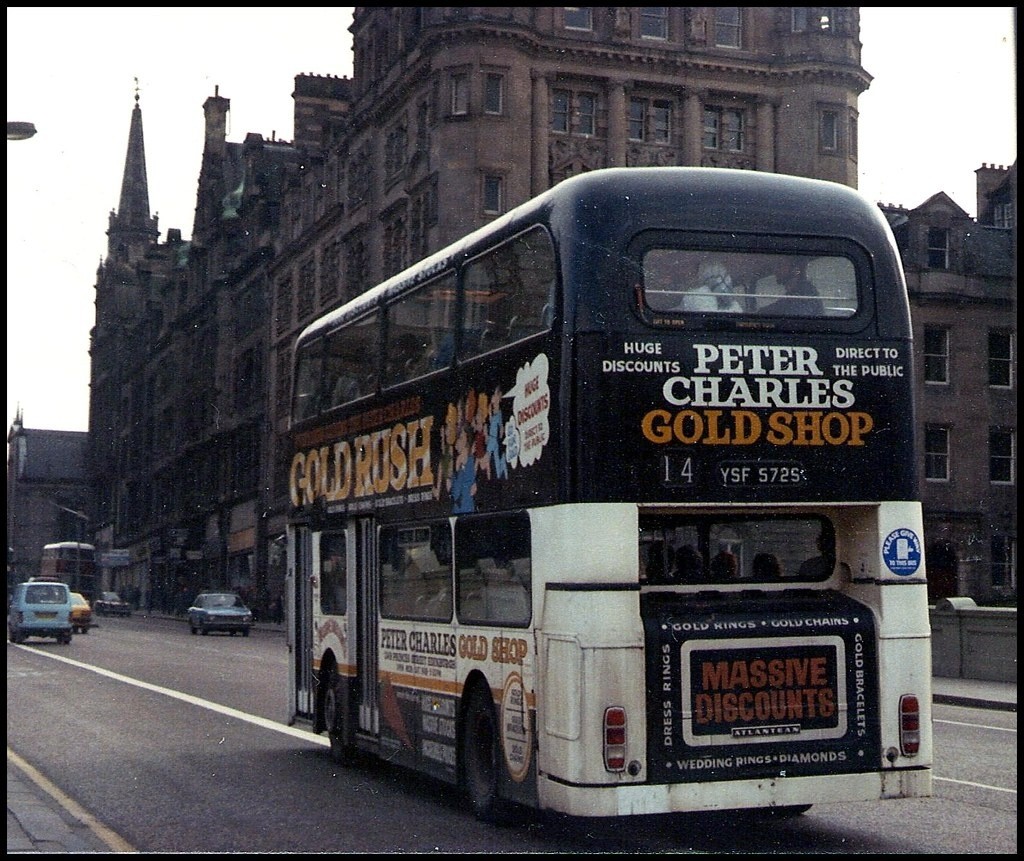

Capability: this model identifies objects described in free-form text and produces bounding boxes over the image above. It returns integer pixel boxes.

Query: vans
[9,577,72,644]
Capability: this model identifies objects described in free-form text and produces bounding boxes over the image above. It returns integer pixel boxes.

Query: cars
[94,592,131,617]
[187,594,254,637]
[41,593,91,634]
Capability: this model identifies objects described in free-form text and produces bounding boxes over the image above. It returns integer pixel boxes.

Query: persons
[119,581,282,623]
[302,260,825,419]
[328,541,824,614]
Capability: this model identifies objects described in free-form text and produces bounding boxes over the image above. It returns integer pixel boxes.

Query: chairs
[414,596,430,616]
[509,557,532,580]
[478,559,497,569]
[485,577,529,622]
[482,569,506,587]
[464,592,483,622]
[430,588,452,618]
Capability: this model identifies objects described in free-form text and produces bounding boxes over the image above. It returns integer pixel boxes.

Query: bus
[287,166,932,820]
